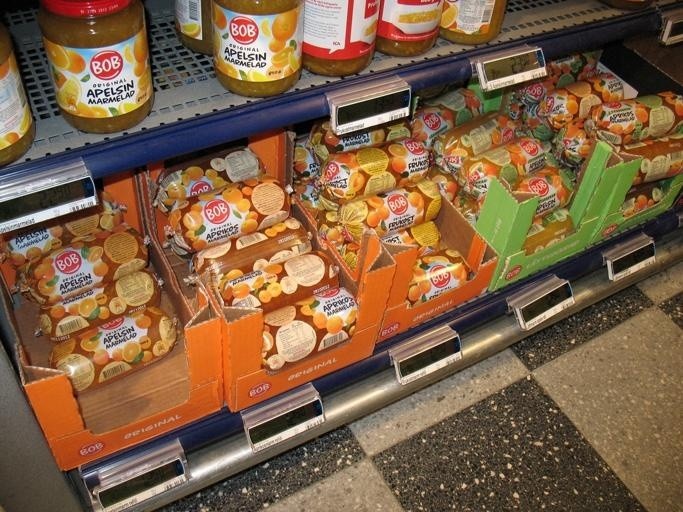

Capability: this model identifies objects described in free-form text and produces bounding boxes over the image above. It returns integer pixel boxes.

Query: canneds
[439,0,509,45]
[302,1,380,76]
[377,0,444,57]
[173,0,214,55]
[1,22,37,167]
[211,0,305,97]
[37,1,155,134]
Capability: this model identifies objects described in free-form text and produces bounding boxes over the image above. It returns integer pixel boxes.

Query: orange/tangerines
[7,55,683,367]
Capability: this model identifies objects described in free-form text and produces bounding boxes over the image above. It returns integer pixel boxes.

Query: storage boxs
[0,1,683,512]
